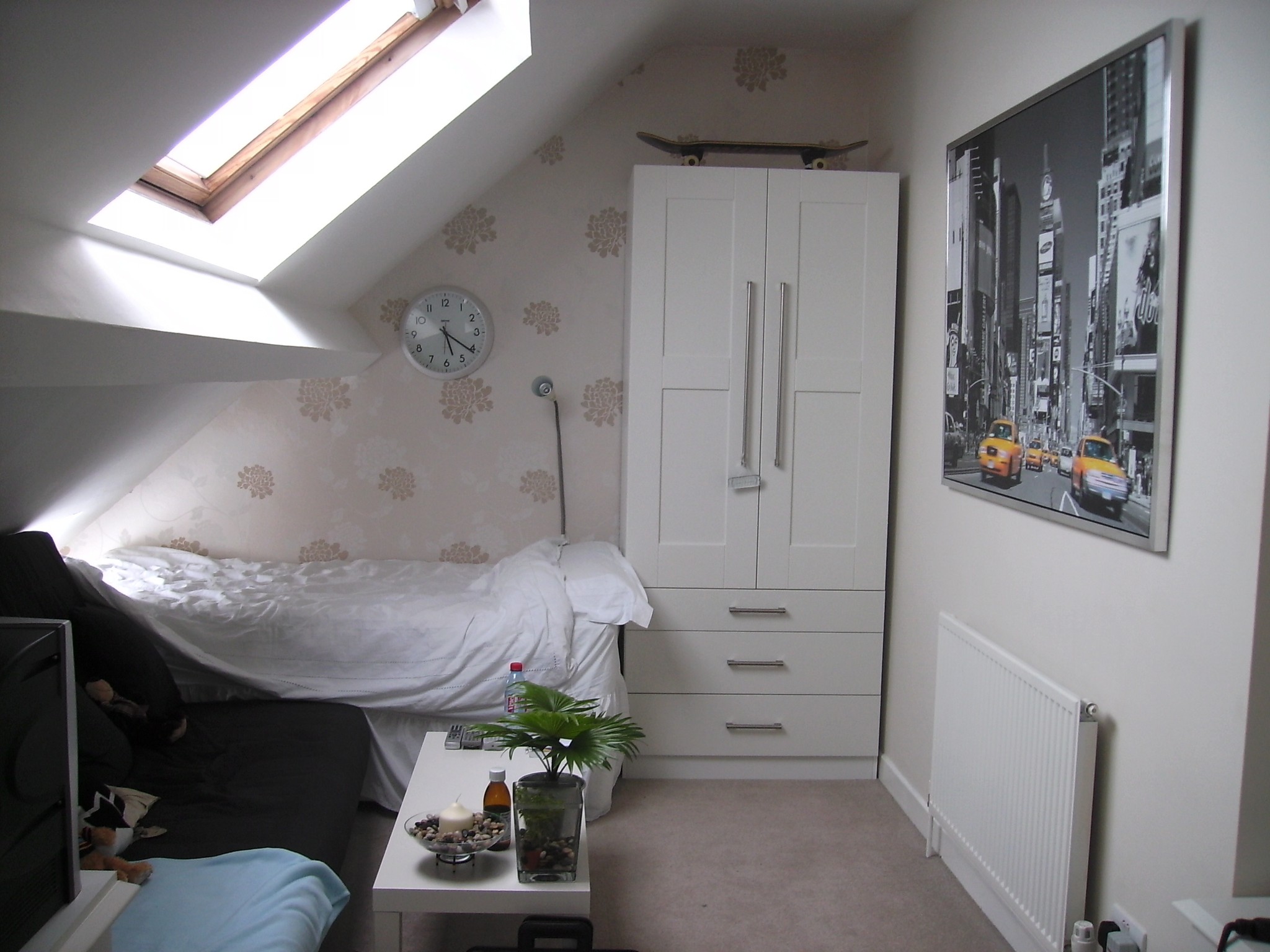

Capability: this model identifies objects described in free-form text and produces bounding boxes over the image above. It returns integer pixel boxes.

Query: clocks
[400,284,495,383]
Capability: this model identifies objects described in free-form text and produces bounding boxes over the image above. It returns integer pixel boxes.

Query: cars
[1043,449,1059,466]
[1070,436,1129,521]
[1025,440,1044,472]
[977,419,1025,487]
[945,411,965,468]
[1057,447,1074,476]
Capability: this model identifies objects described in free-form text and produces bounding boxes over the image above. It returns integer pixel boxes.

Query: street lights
[965,378,989,452]
[1071,349,1125,465]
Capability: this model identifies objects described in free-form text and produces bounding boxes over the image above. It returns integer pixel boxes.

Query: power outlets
[1109,904,1147,952]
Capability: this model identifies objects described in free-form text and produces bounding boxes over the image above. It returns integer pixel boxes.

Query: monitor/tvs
[0,617,81,952]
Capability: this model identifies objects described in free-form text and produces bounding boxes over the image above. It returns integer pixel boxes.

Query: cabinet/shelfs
[613,156,902,789]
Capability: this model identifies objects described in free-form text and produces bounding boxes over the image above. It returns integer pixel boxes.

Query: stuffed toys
[78,826,153,884]
[84,673,187,742]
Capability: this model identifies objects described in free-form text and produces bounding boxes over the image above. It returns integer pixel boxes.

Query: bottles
[505,662,529,721]
[483,766,513,852]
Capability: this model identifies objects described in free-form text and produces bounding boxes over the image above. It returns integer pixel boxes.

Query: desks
[368,730,592,952]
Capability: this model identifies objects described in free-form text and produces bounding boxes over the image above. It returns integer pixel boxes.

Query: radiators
[918,606,1106,952]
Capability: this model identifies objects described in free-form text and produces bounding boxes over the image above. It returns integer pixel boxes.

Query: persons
[1053,302,1060,334]
[1116,217,1161,354]
[1117,459,1153,499]
[945,323,960,397]
[961,430,985,460]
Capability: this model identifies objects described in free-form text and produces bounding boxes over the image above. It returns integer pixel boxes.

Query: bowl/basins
[404,808,509,856]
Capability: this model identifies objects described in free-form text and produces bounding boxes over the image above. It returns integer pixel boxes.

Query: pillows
[559,538,654,631]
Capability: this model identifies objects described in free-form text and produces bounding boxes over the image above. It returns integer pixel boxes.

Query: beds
[69,537,653,826]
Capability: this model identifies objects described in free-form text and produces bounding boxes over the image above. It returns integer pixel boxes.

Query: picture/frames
[938,14,1190,557]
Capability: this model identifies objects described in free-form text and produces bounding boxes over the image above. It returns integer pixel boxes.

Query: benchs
[0,523,370,952]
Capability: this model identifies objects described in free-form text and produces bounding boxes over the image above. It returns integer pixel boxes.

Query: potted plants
[460,671,647,842]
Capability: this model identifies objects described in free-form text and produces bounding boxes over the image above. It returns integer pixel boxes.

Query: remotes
[484,729,504,751]
[462,723,483,749]
[444,724,465,749]
